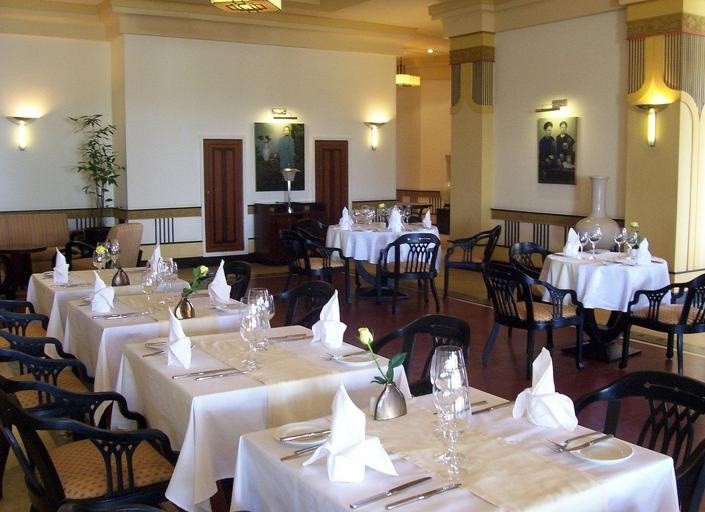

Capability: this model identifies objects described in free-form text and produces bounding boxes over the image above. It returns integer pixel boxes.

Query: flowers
[628,219,640,237]
[94,245,123,267]
[180,265,214,298]
[356,326,405,383]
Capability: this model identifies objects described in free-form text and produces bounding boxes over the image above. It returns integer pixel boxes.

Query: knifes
[348,474,434,509]
[269,333,316,343]
[171,367,247,383]
[472,400,515,419]
[565,433,613,456]
[93,311,152,322]
[383,483,468,512]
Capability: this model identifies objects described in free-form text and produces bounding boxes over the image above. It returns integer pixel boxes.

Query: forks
[545,428,609,448]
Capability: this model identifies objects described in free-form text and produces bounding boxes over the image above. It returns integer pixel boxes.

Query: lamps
[396,56,421,88]
[632,73,678,147]
[271,107,297,125]
[209,0,282,16]
[4,104,44,152]
[280,167,301,214]
[361,105,390,151]
[535,99,567,119]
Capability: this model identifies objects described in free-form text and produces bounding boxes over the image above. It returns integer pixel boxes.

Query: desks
[112,323,414,511]
[324,223,441,305]
[26,266,258,427]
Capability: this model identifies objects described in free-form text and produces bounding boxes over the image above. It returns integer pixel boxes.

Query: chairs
[1,292,182,512]
[571,370,705,512]
[370,313,473,400]
[270,279,333,326]
[444,225,503,299]
[507,240,577,349]
[277,218,352,299]
[478,263,586,382]
[222,259,252,302]
[618,268,705,376]
[375,232,442,314]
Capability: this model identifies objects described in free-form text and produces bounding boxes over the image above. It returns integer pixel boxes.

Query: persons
[254,126,296,191]
[539,121,575,184]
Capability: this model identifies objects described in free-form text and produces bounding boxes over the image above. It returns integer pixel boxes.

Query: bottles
[368,396,378,415]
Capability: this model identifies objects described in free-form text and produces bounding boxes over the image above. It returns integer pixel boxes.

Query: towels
[637,237,651,265]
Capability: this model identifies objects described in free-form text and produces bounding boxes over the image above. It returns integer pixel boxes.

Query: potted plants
[66,111,126,253]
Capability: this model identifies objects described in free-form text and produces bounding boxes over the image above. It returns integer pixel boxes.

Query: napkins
[562,226,583,256]
[422,209,433,229]
[636,237,654,266]
[387,204,404,232]
[338,206,354,230]
[161,305,195,370]
[509,344,581,434]
[89,268,116,313]
[301,381,398,484]
[49,245,72,286]
[309,289,349,354]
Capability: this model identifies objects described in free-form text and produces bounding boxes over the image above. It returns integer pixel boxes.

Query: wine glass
[563,222,663,267]
[427,345,475,490]
[337,204,437,232]
[90,236,122,271]
[136,256,179,310]
[237,287,276,370]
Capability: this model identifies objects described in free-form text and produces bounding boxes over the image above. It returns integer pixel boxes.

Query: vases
[439,155,452,205]
[575,175,620,251]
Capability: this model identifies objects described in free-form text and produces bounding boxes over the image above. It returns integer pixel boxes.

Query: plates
[568,436,636,465]
[329,349,381,369]
[144,335,193,354]
[270,419,334,449]
[216,299,250,315]
